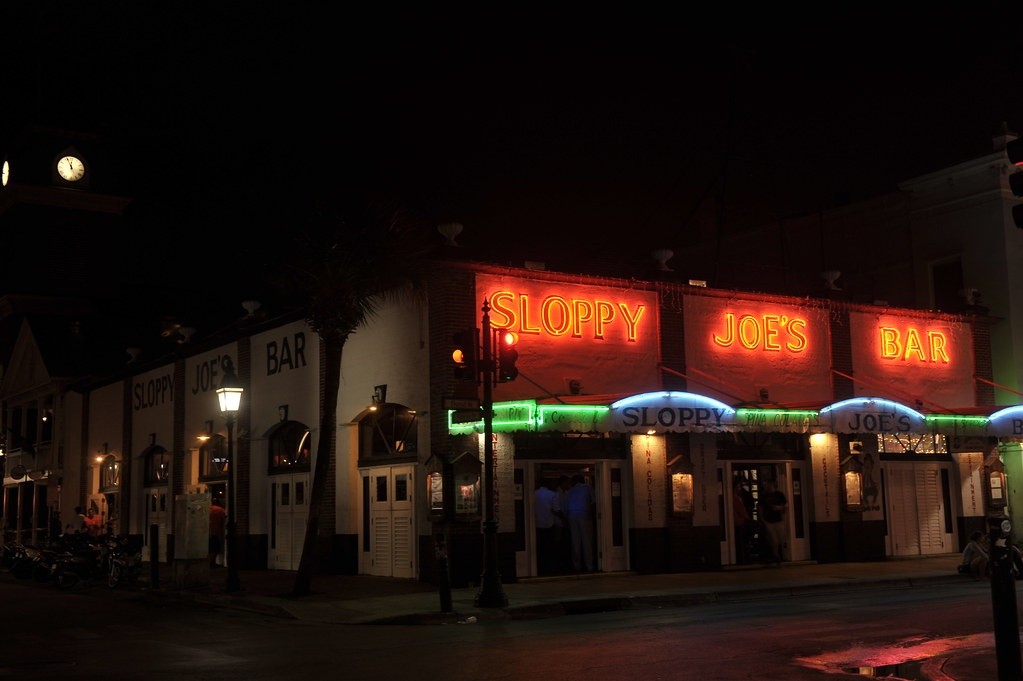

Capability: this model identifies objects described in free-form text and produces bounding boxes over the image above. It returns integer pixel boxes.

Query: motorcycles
[0,522,144,590]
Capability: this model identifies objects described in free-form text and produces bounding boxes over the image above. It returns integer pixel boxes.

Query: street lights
[214,362,245,595]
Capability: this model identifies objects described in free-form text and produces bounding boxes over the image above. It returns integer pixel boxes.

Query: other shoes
[209,562,221,569]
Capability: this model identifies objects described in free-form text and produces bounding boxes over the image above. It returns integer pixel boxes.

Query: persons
[963,531,1023,581]
[734,480,755,563]
[53,511,62,542]
[534,474,596,574]
[759,479,788,561]
[105,502,114,536]
[72,506,101,540]
[209,498,225,568]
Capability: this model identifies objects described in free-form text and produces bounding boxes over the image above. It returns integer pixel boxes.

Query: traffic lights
[453,329,477,373]
[1005,131,1023,229]
[498,329,519,382]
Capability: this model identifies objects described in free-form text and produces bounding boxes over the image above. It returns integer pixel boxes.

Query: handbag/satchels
[958,561,972,572]
[763,507,783,523]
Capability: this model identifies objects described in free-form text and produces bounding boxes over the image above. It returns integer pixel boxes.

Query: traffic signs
[451,412,481,422]
[442,398,480,410]
